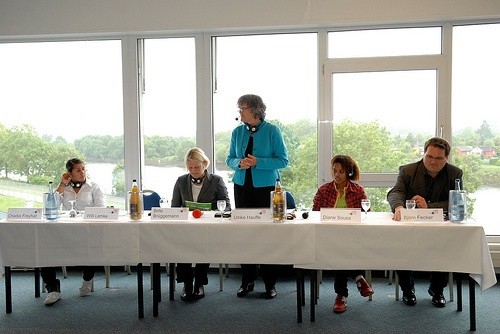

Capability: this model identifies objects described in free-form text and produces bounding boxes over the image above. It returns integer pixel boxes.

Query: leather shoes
[428,288,446,307]
[237,281,254,297]
[266,288,277,298]
[193,285,205,298]
[402,288,417,306]
[180,285,193,300]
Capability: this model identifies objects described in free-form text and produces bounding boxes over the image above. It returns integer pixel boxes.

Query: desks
[0,208,497,332]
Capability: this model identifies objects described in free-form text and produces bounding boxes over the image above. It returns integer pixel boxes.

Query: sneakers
[79,280,91,296]
[44,292,61,304]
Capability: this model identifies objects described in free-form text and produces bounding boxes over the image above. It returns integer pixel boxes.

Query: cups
[406,200,416,209]
[159,199,168,208]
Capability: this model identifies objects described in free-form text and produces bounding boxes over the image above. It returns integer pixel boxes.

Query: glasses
[238,107,251,112]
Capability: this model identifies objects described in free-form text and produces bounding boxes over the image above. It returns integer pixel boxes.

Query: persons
[312,155,374,312]
[225,95,289,298]
[41,158,105,304]
[171,147,231,301]
[387,138,463,308]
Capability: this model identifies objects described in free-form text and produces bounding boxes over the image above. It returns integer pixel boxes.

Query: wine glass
[361,199,370,223]
[217,200,226,222]
[68,200,77,219]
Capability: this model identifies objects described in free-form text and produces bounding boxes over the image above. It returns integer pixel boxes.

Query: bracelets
[59,181,66,189]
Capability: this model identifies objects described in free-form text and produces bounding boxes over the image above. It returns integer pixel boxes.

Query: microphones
[302,210,309,219]
[235,115,241,120]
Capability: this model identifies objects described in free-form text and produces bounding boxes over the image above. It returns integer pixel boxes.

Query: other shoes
[356,278,374,298]
[333,294,347,311]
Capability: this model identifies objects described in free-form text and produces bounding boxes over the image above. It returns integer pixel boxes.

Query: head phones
[244,119,263,132]
[191,175,205,183]
[349,165,355,177]
[70,178,86,187]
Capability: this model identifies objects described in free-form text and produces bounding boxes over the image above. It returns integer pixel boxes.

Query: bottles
[44,182,58,222]
[129,179,142,221]
[271,179,287,223]
[450,179,465,224]
